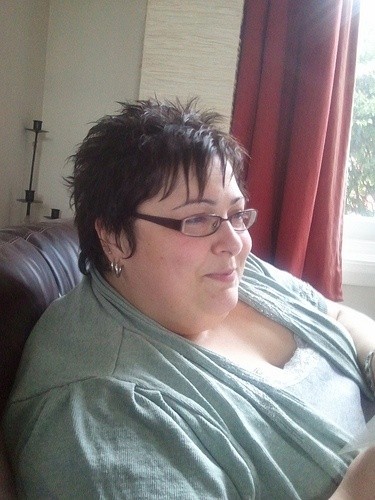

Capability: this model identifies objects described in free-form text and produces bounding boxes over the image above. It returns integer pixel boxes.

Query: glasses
[130,209,257,237]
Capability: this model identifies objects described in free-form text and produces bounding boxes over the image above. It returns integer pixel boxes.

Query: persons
[2,95,375,500]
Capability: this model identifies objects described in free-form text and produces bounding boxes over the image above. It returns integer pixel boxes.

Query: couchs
[1,219,92,420]
